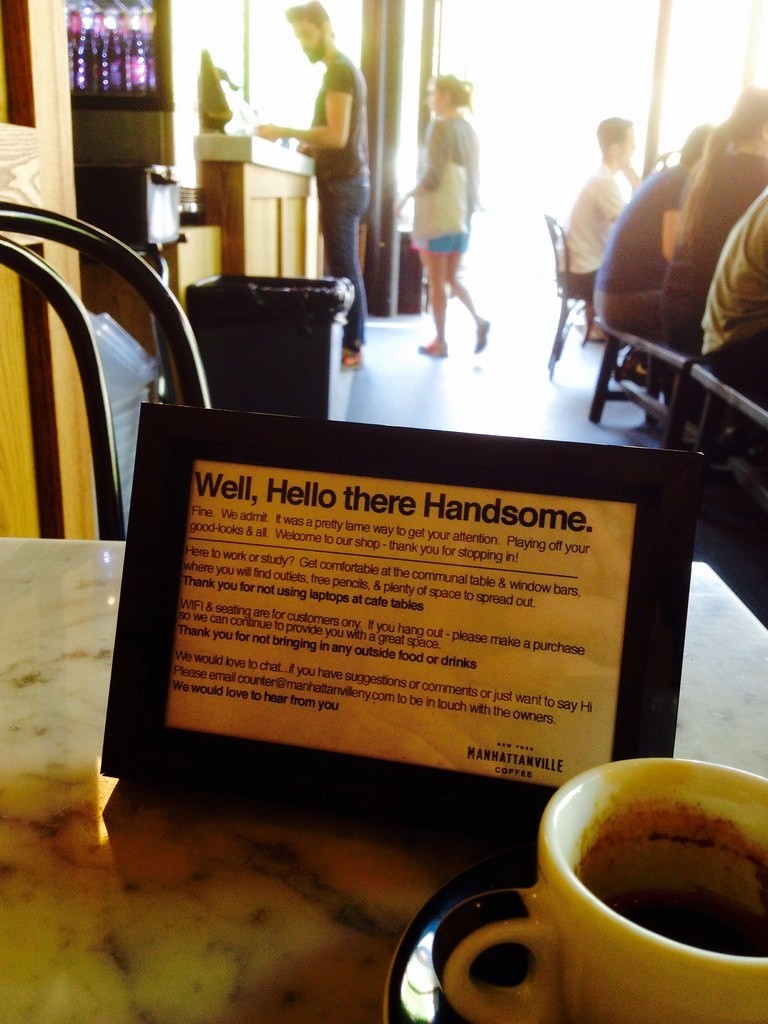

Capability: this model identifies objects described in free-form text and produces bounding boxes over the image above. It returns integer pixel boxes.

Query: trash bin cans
[182,272,353,420]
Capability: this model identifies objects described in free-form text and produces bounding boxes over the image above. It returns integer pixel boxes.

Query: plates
[384,844,536,1024]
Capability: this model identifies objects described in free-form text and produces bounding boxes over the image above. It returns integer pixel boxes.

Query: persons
[394,74,491,358]
[658,84,768,449]
[591,122,717,391]
[254,0,370,367]
[556,117,644,300]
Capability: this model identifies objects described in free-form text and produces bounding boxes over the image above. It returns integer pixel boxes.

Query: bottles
[126,18,149,90]
[110,16,126,91]
[88,13,110,90]
[67,10,88,90]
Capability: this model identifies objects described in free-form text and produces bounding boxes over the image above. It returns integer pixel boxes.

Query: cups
[434,759,768,1024]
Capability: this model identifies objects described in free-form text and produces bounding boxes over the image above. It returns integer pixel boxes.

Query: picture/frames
[98,400,706,842]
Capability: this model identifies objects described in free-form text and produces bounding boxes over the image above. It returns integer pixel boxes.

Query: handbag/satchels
[412,123,470,239]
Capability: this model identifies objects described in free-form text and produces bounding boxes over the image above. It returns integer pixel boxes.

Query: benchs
[588,312,768,509]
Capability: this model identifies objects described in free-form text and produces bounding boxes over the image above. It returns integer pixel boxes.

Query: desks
[198,132,320,280]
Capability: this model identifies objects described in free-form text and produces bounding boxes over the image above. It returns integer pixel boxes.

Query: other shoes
[341,347,362,372]
[418,339,448,358]
[474,319,491,352]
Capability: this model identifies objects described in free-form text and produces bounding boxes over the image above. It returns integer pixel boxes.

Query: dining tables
[0,528,767,1023]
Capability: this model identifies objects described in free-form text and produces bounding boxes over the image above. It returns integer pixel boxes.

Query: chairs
[0,201,219,539]
[541,213,605,378]
